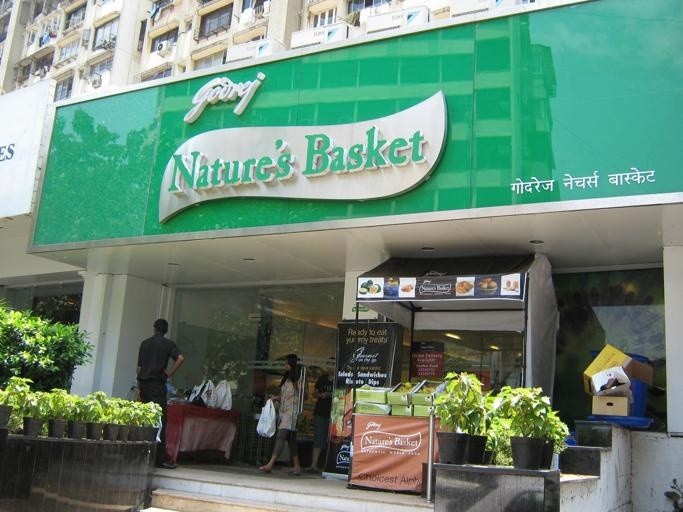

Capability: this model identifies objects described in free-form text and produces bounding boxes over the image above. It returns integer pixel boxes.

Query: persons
[258,354,309,476]
[135,319,186,468]
[302,357,336,473]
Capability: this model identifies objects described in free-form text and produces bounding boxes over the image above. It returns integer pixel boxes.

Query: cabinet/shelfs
[246,360,329,467]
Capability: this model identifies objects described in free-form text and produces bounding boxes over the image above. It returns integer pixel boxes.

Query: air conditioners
[91,73,101,87]
[157,41,169,55]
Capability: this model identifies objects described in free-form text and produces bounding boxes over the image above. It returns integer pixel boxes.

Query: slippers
[259,466,320,475]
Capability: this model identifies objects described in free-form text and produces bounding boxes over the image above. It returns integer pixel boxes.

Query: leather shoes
[155,462,175,468]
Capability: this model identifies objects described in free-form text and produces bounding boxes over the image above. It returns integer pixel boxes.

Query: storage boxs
[592,395,631,417]
[355,379,443,417]
[584,344,654,396]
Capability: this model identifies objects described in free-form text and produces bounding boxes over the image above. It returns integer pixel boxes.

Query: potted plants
[541,406,569,468]
[0,377,164,442]
[434,371,472,465]
[494,386,544,470]
[468,395,487,465]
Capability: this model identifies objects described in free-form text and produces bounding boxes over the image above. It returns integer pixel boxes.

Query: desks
[164,400,239,465]
